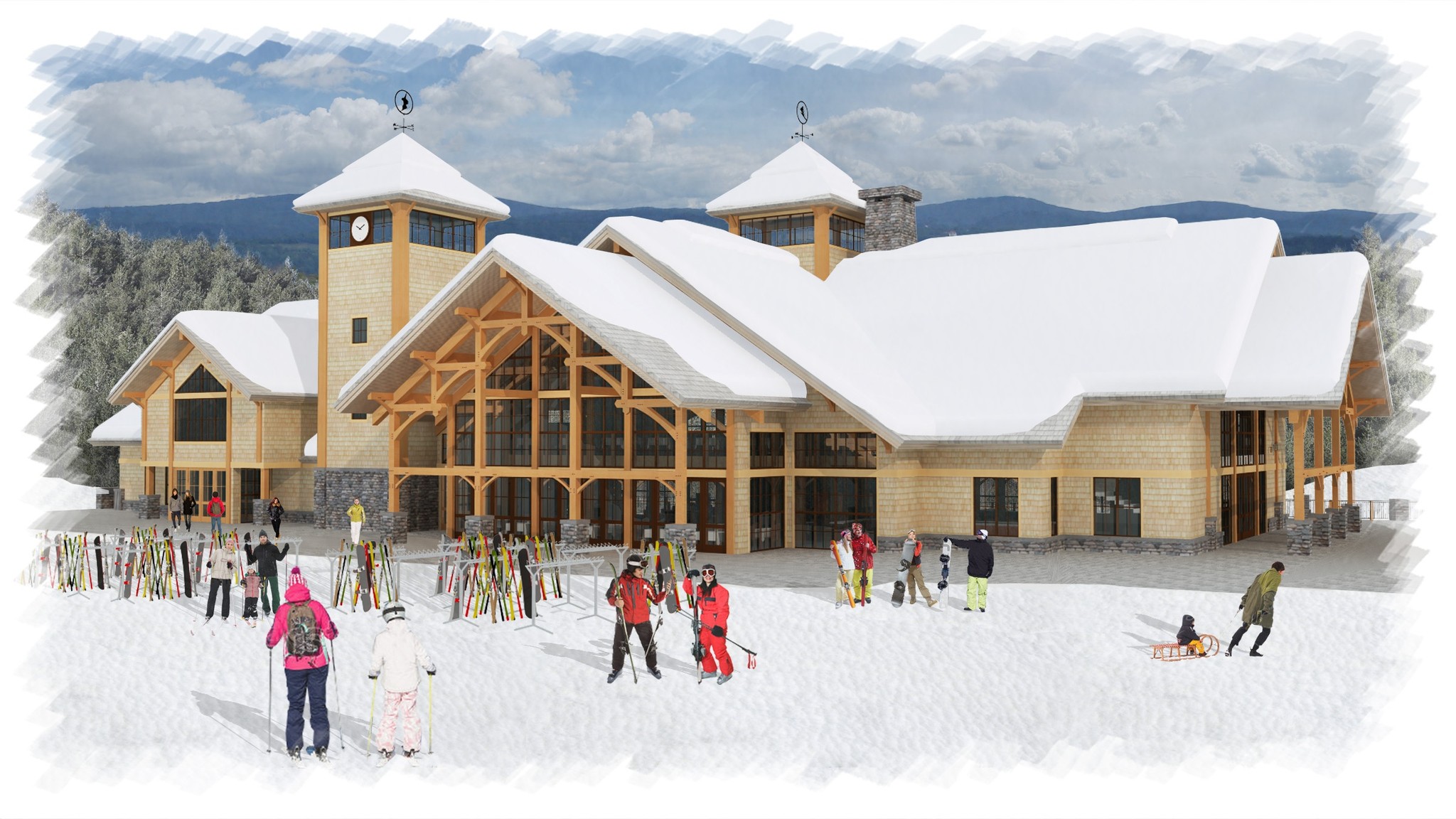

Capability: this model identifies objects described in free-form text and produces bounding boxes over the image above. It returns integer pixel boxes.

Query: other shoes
[287,745,417,759]
[835,596,986,613]
[205,612,276,620]
[174,525,191,533]
[1190,644,1262,657]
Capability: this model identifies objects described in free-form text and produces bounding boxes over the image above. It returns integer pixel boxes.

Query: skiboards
[191,618,227,636]
[609,562,672,684]
[21,524,245,601]
[640,538,694,609]
[830,540,856,608]
[267,617,273,626]
[691,574,702,685]
[374,751,421,767]
[180,541,192,598]
[448,530,563,624]
[245,619,257,629]
[861,533,868,607]
[281,746,336,770]
[333,537,398,610]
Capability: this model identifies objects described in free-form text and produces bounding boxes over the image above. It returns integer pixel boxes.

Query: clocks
[351,216,369,242]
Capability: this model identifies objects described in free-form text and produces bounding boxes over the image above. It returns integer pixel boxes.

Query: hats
[246,565,255,571]
[259,529,267,538]
[224,538,234,545]
[286,566,308,588]
[839,522,988,538]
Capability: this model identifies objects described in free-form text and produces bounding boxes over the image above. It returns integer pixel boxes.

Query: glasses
[977,530,982,535]
[908,529,916,533]
[702,569,715,577]
[640,561,649,568]
[853,523,861,528]
[845,529,851,533]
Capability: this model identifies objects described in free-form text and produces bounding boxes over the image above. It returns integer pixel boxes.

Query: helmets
[382,602,406,622]
[627,554,644,573]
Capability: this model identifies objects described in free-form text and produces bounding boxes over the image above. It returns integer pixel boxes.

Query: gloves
[943,536,949,542]
[687,569,700,578]
[284,543,290,551]
[241,579,265,585]
[986,572,992,578]
[206,561,232,568]
[244,532,250,539]
[712,626,723,636]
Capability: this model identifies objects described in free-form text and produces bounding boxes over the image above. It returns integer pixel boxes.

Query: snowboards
[493,534,507,594]
[518,549,536,619]
[124,542,137,598]
[452,550,470,620]
[939,539,952,609]
[659,546,678,614]
[39,536,51,583]
[163,528,173,577]
[196,533,206,583]
[437,536,452,594]
[526,539,541,603]
[652,542,669,606]
[115,528,125,577]
[366,541,376,595]
[891,539,916,608]
[356,545,372,612]
[54,534,65,586]
[244,532,253,570]
[94,536,105,590]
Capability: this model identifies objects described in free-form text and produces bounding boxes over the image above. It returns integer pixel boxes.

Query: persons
[347,497,366,549]
[1225,561,1285,657]
[207,491,225,538]
[905,529,937,607]
[943,529,993,612]
[240,563,267,620]
[266,566,339,763]
[182,490,196,532]
[204,539,238,620]
[1176,614,1207,657]
[830,529,856,609]
[837,523,877,603]
[169,488,183,532]
[266,497,284,540]
[244,529,290,617]
[606,554,671,682]
[368,600,437,759]
[682,564,734,685]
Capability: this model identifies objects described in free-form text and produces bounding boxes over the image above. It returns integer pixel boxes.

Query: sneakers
[607,669,731,684]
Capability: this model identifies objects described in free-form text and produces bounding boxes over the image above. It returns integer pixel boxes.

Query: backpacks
[284,600,320,655]
[211,500,221,515]
